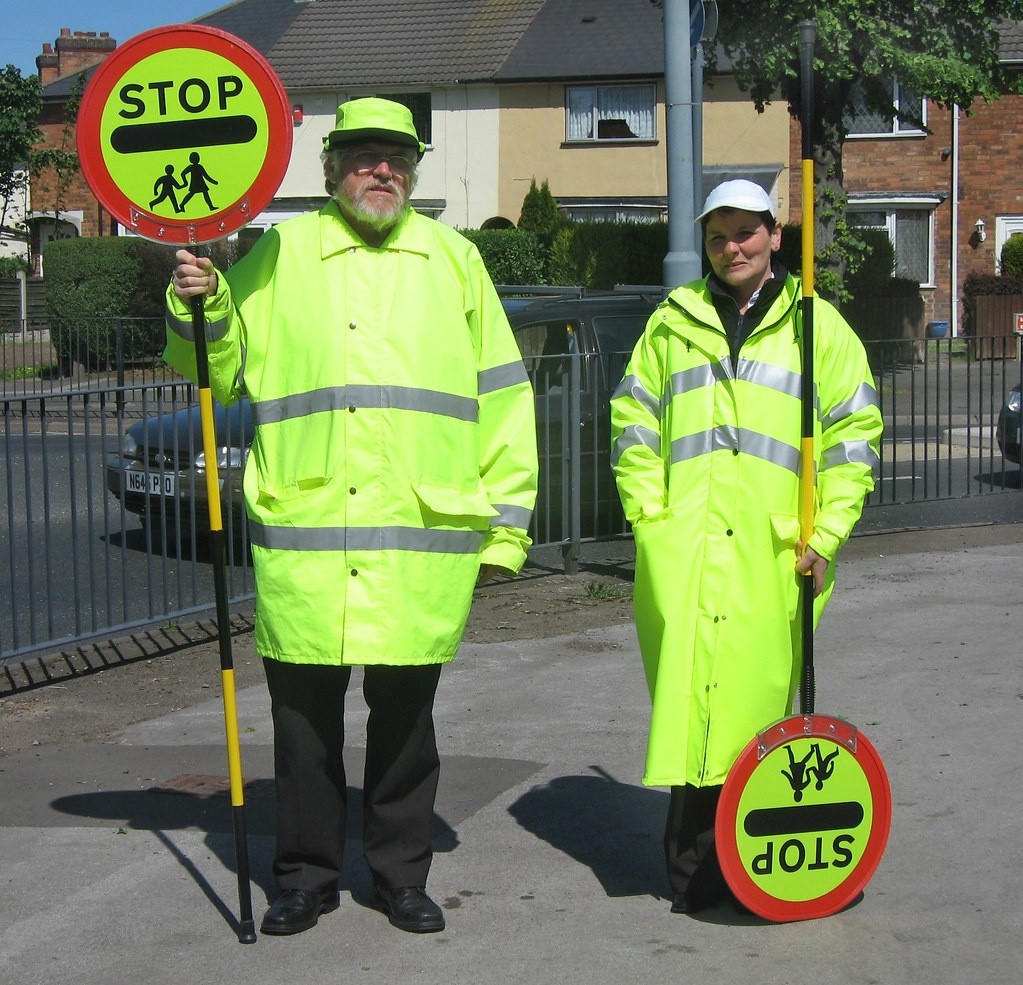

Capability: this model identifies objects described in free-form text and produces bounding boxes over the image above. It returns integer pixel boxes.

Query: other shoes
[670,895,713,913]
[731,898,753,914]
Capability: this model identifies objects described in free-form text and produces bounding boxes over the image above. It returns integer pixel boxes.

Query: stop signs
[714,712,891,922]
[78,25,294,249]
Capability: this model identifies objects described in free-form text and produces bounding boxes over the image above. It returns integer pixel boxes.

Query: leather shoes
[369,884,445,932]
[260,882,340,934]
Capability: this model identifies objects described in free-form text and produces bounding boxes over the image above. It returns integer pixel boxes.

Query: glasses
[341,150,418,176]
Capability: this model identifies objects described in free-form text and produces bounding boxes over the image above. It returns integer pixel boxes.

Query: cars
[996,384,1020,464]
[107,293,670,542]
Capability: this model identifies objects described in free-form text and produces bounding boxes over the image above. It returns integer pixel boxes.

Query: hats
[322,97,426,163]
[694,178,778,223]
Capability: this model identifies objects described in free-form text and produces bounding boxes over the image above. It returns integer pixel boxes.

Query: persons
[161,97,538,933]
[609,180,884,914]
